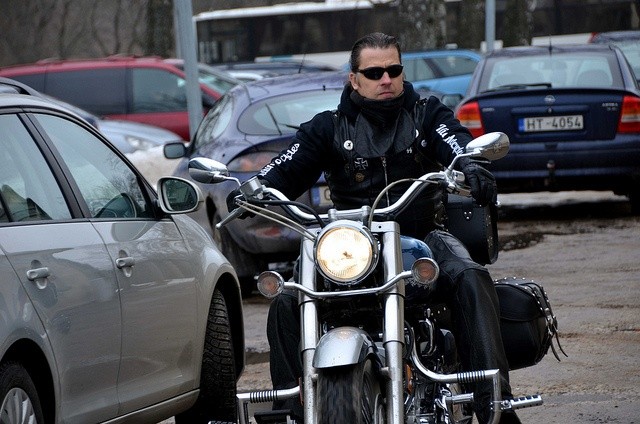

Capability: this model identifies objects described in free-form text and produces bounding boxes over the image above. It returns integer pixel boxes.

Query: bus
[183,0,375,66]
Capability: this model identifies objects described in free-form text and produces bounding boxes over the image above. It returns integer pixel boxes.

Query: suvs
[0,57,245,142]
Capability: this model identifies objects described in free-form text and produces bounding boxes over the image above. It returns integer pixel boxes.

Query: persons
[226,31,522,424]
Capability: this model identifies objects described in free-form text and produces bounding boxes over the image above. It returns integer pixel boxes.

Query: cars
[452,44,639,222]
[165,69,352,279]
[338,51,483,103]
[1,94,246,419]
[0,75,186,189]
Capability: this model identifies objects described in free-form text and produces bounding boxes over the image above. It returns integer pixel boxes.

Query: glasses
[356,64,404,80]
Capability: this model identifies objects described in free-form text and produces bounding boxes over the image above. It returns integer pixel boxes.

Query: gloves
[226,189,249,219]
[461,156,499,209]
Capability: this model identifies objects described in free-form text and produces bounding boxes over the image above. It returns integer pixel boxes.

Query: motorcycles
[185,132,543,417]
